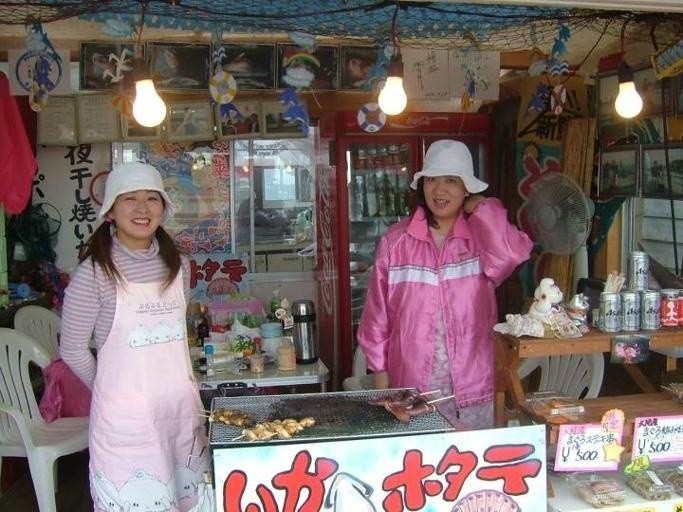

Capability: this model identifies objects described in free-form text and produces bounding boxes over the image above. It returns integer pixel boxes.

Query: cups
[292,300,318,364]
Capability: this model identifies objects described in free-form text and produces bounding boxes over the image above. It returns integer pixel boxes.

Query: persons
[356,139,534,430]
[58,163,212,512]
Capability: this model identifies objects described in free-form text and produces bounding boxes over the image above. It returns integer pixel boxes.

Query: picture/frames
[640,142,683,201]
[600,144,639,199]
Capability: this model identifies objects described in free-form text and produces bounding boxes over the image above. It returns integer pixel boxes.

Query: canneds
[642,290,662,330]
[620,291,641,331]
[661,289,680,329]
[679,289,683,328]
[627,250,651,290]
[600,293,621,334]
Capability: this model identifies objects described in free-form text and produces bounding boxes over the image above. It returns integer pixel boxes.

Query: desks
[491,321,683,498]
[198,358,331,392]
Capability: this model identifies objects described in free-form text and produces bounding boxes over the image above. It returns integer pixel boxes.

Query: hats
[409,140,489,194]
[99,163,176,227]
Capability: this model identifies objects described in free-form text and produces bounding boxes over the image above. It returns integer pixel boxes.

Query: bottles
[251,338,264,374]
[199,357,208,379]
[373,168,386,216]
[384,167,399,216]
[354,175,366,217]
[198,305,210,346]
[395,165,411,217]
[205,346,216,376]
[346,144,410,168]
[364,168,379,217]
[271,290,282,318]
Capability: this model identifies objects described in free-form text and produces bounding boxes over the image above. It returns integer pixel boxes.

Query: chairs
[0,327,89,512]
[507,351,605,429]
[14,305,97,362]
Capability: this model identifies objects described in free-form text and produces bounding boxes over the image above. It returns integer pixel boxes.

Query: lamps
[131,0,167,129]
[378,6,407,116]
[615,17,643,118]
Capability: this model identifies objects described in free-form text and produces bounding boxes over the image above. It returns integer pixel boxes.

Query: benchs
[517,391,683,425]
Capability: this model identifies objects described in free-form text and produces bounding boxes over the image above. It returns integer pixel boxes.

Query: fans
[526,172,595,255]
[18,202,61,244]
